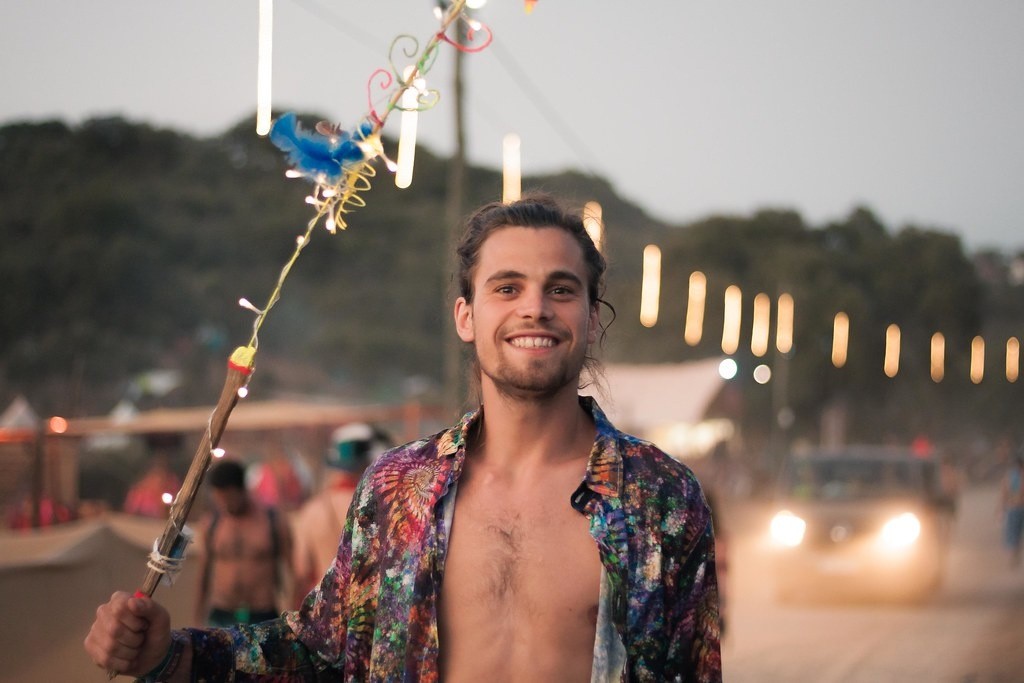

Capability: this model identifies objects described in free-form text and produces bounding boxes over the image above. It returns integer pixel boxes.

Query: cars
[763,445,955,612]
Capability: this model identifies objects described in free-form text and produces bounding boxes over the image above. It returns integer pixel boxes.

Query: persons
[84,197,723,683]
[126,417,390,629]
[938,446,1024,571]
